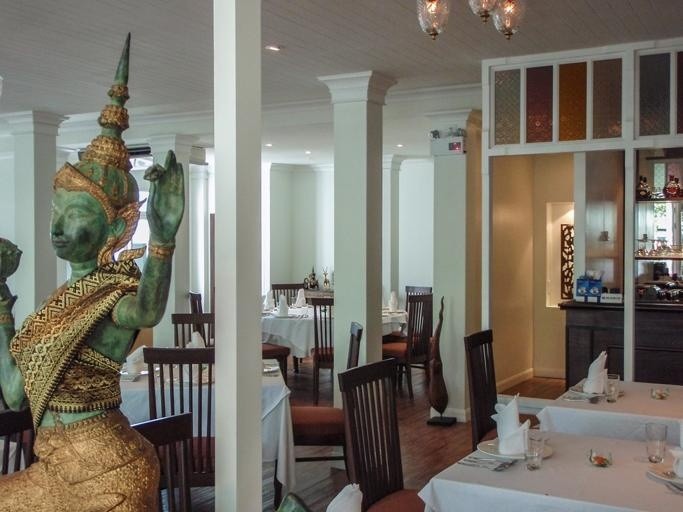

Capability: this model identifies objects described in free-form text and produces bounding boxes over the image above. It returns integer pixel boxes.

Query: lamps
[416,0,522,40]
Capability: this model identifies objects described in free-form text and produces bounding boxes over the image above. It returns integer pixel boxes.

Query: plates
[268,312,295,318]
[477,437,551,459]
[643,463,682,485]
[261,310,273,314]
[570,385,621,397]
[170,374,214,386]
[264,363,278,373]
[672,453,683,478]
[382,306,403,316]
[119,367,159,375]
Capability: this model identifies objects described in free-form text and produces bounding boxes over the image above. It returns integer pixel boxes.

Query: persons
[0,149,185,512]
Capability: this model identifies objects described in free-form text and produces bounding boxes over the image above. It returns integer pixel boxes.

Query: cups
[658,287,680,300]
[651,186,663,198]
[301,304,308,317]
[603,374,619,402]
[523,428,544,471]
[588,448,613,466]
[650,386,669,400]
[644,421,667,461]
[289,296,296,308]
[307,297,312,308]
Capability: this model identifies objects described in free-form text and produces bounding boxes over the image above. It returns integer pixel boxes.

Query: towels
[583,351,610,392]
[325,483,364,511]
[126,345,145,374]
[295,288,306,308]
[490,394,532,458]
[278,295,288,316]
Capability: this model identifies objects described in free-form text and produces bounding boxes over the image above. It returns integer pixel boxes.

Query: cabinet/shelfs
[571,148,683,307]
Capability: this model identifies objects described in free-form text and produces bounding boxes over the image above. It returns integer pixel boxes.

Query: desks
[558,300,683,392]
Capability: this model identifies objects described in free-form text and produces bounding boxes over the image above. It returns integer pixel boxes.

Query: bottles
[636,175,650,199]
[388,293,396,310]
[663,175,680,198]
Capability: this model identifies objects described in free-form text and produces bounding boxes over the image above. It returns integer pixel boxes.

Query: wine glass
[634,238,682,257]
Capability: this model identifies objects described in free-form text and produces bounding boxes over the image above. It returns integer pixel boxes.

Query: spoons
[459,455,517,472]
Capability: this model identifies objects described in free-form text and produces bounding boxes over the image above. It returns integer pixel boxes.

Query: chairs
[273,322,363,511]
[172,291,214,348]
[310,298,334,406]
[262,342,290,390]
[464,329,539,453]
[271,284,305,308]
[1,408,37,476]
[337,356,425,512]
[131,347,215,511]
[381,286,434,399]
